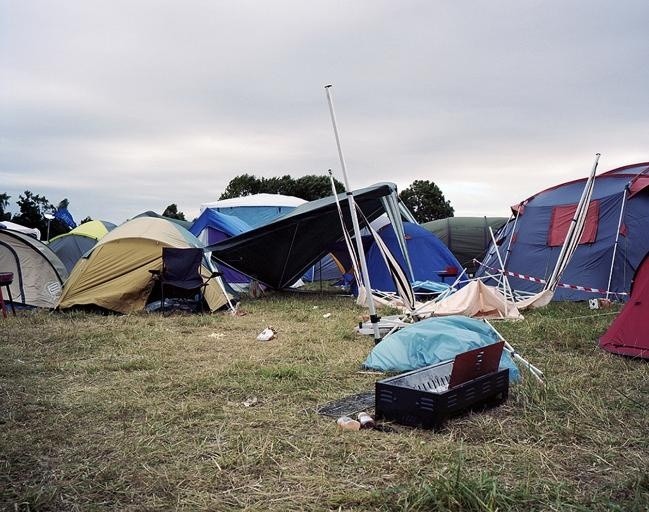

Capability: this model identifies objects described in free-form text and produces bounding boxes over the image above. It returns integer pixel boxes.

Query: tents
[188,206,305,302]
[41,218,119,276]
[200,192,347,283]
[420,214,513,279]
[132,210,194,231]
[54,215,238,318]
[598,255,649,362]
[0,220,69,313]
[331,221,470,305]
[475,162,649,306]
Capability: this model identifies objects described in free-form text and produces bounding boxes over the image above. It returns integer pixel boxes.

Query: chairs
[145,247,224,319]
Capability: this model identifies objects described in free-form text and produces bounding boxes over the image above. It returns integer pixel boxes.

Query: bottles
[336,412,376,432]
